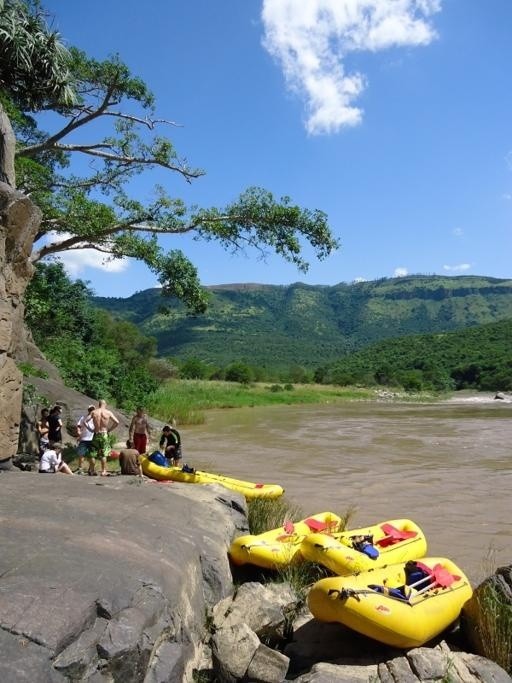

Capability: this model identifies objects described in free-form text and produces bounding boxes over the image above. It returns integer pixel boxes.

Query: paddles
[409,563,453,599]
[283,522,294,535]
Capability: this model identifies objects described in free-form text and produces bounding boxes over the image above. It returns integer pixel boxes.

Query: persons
[159,425,182,467]
[84,399,120,475]
[119,439,143,477]
[36,407,49,461]
[72,404,97,473]
[42,405,63,451]
[129,405,152,456]
[38,442,74,474]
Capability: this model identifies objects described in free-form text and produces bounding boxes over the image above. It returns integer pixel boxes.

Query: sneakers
[72,468,83,473]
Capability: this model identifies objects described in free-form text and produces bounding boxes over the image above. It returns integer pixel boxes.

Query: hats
[52,443,61,447]
[88,405,96,409]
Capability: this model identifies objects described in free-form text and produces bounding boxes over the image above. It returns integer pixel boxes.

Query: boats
[229,512,347,571]
[138,453,285,502]
[308,557,473,648]
[300,519,427,576]
